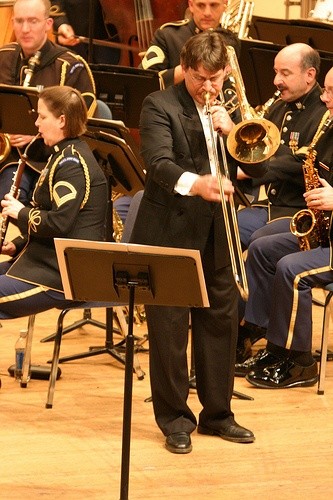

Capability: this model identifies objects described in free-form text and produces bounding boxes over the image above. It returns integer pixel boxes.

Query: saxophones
[106,189,149,346]
[290,108,330,252]
[0,50,41,164]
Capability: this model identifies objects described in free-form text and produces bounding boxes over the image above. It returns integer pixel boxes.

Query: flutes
[0,132,41,254]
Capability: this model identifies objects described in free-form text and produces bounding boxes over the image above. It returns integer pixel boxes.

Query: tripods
[41,119,255,402]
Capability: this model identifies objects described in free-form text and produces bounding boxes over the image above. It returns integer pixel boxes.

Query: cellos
[98,0,189,156]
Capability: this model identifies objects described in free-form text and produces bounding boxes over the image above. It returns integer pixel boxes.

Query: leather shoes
[233,348,292,378]
[245,356,319,389]
[197,414,256,443]
[165,431,192,454]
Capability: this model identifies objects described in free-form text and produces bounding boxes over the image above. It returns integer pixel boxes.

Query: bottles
[14,330,32,383]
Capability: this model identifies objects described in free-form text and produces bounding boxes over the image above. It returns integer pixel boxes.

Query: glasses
[14,16,49,26]
[187,68,225,86]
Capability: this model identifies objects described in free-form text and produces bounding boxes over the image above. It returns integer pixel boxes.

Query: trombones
[202,45,281,301]
[221,0,254,38]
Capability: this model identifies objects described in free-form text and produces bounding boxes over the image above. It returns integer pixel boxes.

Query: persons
[235,67,333,388]
[237,42,327,252]
[139,0,229,91]
[0,86,111,320]
[50,0,120,65]
[130,32,254,454]
[0,0,95,207]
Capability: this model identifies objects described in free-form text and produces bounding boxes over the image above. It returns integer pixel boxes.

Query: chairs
[22,303,127,409]
[311,283,333,395]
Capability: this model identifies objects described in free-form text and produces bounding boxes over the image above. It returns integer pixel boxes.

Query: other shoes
[236,324,267,364]
